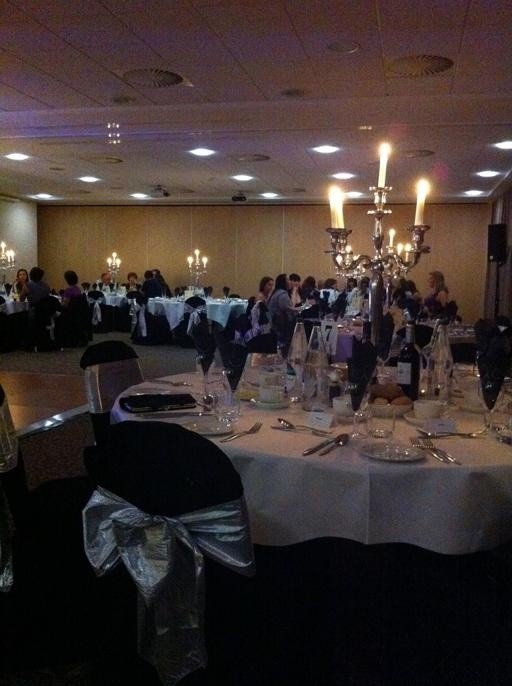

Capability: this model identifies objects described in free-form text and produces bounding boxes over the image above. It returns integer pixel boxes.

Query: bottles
[398,324,419,401]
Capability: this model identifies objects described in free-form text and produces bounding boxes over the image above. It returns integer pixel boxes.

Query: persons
[345,277,368,319]
[19,267,50,320]
[270,272,305,344]
[143,270,161,309]
[9,269,29,301]
[289,273,304,308]
[323,278,341,307]
[305,274,318,320]
[152,268,171,297]
[381,275,421,360]
[253,277,274,306]
[424,272,449,324]
[51,271,81,323]
[120,272,146,298]
[96,273,116,301]
[335,279,357,318]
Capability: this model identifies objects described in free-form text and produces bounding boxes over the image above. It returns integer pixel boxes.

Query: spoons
[278,418,330,434]
[318,433,350,456]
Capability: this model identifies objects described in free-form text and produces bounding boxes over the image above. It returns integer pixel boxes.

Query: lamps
[105,121,124,146]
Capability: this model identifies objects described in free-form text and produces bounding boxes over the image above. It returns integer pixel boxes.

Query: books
[119,394,197,412]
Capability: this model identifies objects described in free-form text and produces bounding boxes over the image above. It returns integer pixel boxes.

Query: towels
[347,333,377,413]
[215,326,248,393]
[474,317,512,410]
[189,312,222,378]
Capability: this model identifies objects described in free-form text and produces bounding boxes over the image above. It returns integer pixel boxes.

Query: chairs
[80,340,144,425]
[390,325,438,366]
[239,297,255,343]
[247,302,277,352]
[0,417,250,686]
[404,299,420,316]
[174,287,187,296]
[183,297,208,349]
[364,312,395,357]
[203,286,212,296]
[85,291,109,334]
[296,318,321,350]
[127,291,172,344]
[434,301,456,323]
[61,293,90,346]
[4,283,12,294]
[26,296,61,347]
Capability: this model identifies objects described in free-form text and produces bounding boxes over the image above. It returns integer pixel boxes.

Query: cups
[354,404,395,438]
[216,390,241,422]
[332,397,348,415]
[259,385,285,403]
[474,379,506,437]
[413,399,447,420]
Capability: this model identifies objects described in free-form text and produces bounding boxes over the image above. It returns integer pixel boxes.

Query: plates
[403,413,444,425]
[368,402,413,417]
[170,416,238,437]
[359,441,428,462]
[250,396,291,410]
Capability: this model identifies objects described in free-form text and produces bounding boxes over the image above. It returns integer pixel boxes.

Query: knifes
[303,436,338,456]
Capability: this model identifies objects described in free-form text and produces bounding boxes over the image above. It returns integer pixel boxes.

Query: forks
[415,429,465,438]
[221,422,263,443]
[422,437,463,466]
[409,437,445,463]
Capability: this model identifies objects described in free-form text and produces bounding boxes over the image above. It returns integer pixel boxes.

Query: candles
[202,256,208,268]
[107,257,112,266]
[188,256,193,265]
[405,242,412,262]
[112,252,117,263]
[1,243,4,253]
[330,184,343,230]
[378,142,390,190]
[389,229,395,247]
[116,259,120,267]
[195,249,200,259]
[415,179,430,224]
[397,243,403,258]
[10,249,15,261]
[6,251,11,262]
